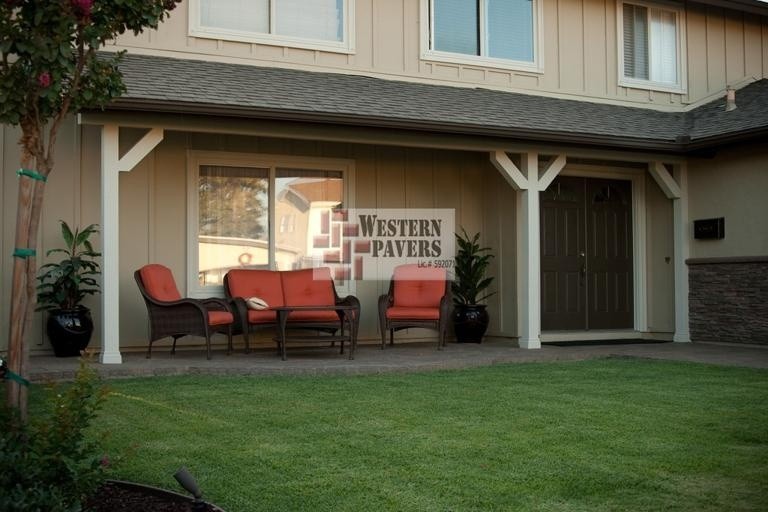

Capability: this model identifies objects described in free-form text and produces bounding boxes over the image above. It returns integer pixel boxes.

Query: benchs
[223,269,360,354]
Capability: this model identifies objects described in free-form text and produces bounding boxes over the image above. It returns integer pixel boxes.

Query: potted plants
[448,226,496,344]
[38,220,101,356]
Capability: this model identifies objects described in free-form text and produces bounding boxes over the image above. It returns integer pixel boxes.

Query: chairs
[135,265,233,360]
[378,263,450,350]
